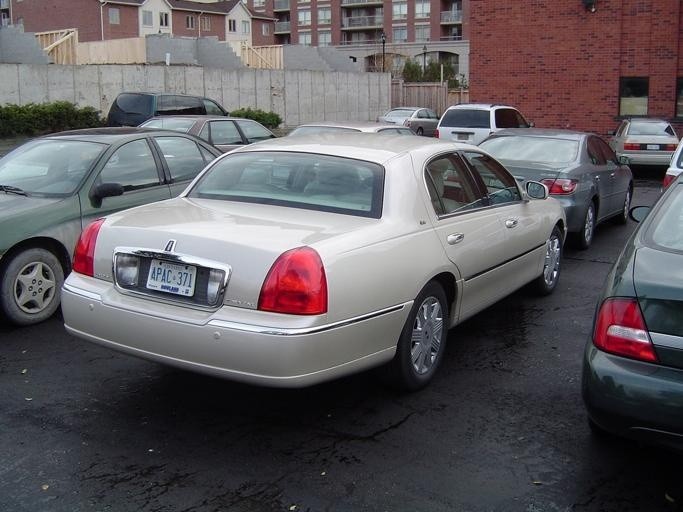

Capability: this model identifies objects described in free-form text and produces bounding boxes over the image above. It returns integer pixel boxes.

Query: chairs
[303,157,460,215]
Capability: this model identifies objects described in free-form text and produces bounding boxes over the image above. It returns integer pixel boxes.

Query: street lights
[381,32,386,72]
[422,45,427,71]
